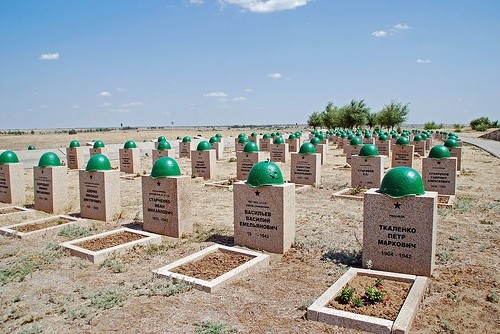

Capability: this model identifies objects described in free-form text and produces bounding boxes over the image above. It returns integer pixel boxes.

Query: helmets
[428,145,450,161]
[86,154,113,172]
[176,136,181,140]
[158,141,171,150]
[358,143,379,158]
[124,140,136,149]
[151,157,182,179]
[197,141,211,150]
[243,142,259,153]
[247,159,284,188]
[396,137,410,146]
[376,166,424,198]
[182,127,460,150]
[70,140,80,148]
[38,152,62,167]
[298,142,316,156]
[0,150,20,165]
[158,135,167,142]
[93,140,104,148]
[28,145,37,150]
